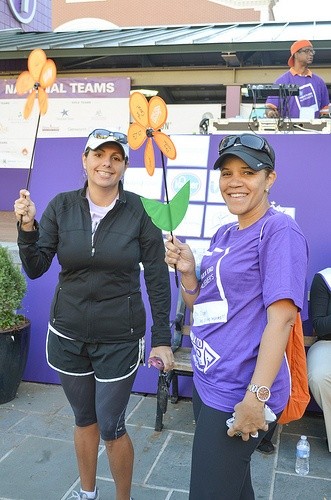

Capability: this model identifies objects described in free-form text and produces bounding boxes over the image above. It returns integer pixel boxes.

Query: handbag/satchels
[222,215,309,423]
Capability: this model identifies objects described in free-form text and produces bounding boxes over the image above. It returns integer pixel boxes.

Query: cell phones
[147,357,164,371]
[227,404,276,436]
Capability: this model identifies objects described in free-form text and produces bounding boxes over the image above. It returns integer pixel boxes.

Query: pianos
[208,82,331,135]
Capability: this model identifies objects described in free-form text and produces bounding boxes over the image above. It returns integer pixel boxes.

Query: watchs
[246,383,271,402]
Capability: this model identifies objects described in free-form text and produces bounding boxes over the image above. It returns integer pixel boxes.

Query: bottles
[295,436,310,475]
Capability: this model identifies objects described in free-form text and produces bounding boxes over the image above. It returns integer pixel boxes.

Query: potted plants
[0,245,32,405]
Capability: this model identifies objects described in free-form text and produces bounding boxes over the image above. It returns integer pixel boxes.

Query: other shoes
[256,441,274,453]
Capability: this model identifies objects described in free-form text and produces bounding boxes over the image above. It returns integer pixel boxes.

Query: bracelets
[180,280,200,295]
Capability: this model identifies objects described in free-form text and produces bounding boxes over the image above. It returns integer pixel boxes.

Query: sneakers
[67,486,100,500]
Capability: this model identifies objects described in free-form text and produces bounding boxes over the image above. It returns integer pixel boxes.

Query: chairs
[155,289,194,431]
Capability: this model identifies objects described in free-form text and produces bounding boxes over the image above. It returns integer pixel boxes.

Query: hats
[85,133,129,160]
[287,40,312,66]
[213,136,276,172]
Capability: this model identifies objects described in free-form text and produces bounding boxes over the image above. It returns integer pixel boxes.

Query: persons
[257,266,331,454]
[264,40,330,118]
[14,128,176,500]
[164,135,309,500]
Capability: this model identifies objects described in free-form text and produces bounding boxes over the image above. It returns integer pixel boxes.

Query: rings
[172,361,174,362]
[251,431,258,438]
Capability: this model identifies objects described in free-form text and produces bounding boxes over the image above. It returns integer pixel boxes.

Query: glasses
[93,129,128,145]
[299,49,316,56]
[219,134,264,151]
